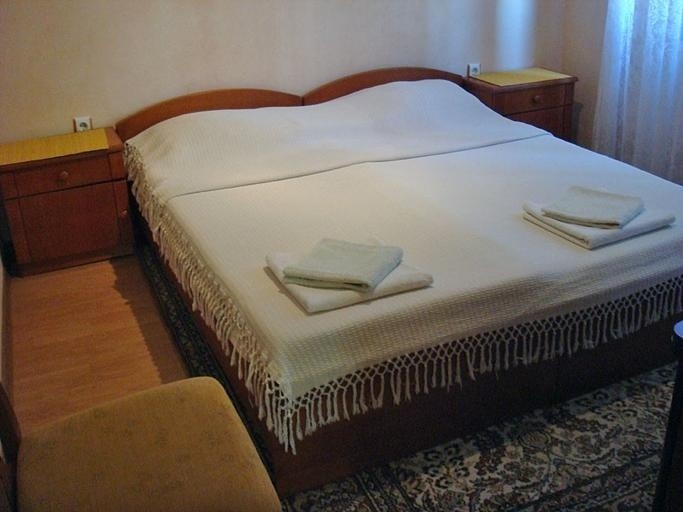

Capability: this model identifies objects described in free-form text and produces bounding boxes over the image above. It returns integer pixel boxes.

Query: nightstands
[469,62,577,144]
[0,129,135,277]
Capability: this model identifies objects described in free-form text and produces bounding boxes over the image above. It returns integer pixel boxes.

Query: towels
[546,179,644,232]
[278,237,406,290]
[520,188,676,249]
[264,234,435,316]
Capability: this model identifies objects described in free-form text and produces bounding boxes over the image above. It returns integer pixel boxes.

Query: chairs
[0,374,288,511]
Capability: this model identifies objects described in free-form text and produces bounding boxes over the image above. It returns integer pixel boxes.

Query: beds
[112,62,681,502]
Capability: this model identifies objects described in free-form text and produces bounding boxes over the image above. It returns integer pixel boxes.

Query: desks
[648,318,682,512]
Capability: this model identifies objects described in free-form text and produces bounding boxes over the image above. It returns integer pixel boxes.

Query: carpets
[126,246,682,512]
